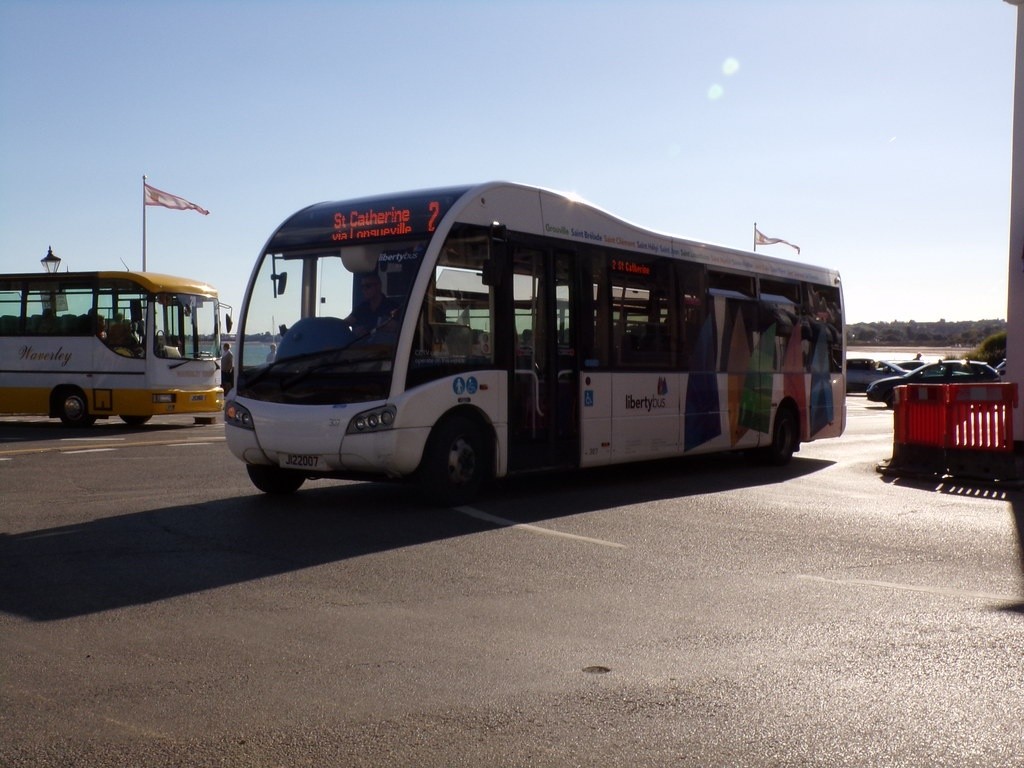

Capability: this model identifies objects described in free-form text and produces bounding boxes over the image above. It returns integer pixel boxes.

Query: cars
[866,356,1007,409]
[846,357,967,393]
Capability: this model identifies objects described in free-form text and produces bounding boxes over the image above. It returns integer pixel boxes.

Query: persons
[343,276,388,336]
[109,313,132,346]
[962,357,972,371]
[267,342,277,362]
[221,343,233,397]
[913,352,923,360]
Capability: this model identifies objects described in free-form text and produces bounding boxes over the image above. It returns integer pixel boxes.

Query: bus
[0,271,233,426]
[224,180,846,507]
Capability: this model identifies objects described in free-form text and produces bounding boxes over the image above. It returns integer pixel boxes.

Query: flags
[143,183,209,216]
[755,228,800,254]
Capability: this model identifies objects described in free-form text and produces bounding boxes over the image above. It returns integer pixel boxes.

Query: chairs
[0,314,146,354]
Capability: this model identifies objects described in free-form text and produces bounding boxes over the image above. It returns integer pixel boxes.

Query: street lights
[41,245,61,318]
